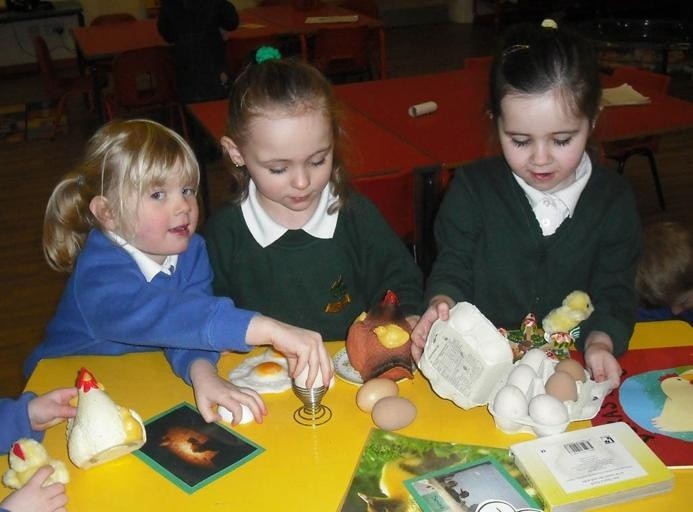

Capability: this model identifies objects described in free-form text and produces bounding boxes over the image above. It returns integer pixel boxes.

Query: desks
[0,319,692,511]
[330,65,693,236]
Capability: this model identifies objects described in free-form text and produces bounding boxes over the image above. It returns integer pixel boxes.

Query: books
[403,451,541,511]
[340,427,546,511]
[511,420,676,512]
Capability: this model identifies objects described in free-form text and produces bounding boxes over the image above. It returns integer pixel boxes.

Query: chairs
[36,2,393,136]
[182,59,691,264]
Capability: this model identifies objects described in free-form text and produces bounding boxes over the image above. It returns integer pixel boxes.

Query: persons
[1,379,85,512]
[624,217,693,331]
[154,1,241,103]
[182,39,425,350]
[13,115,338,431]
[407,8,650,399]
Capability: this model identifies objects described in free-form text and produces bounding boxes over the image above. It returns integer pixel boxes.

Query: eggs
[520,347,547,374]
[544,371,577,402]
[294,362,326,389]
[228,345,293,394]
[554,359,587,383]
[493,385,528,421]
[370,396,416,431]
[528,394,569,423]
[355,377,398,413]
[506,364,536,393]
[218,403,254,425]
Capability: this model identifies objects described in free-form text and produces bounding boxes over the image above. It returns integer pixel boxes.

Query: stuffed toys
[541,290,596,336]
[3,436,71,491]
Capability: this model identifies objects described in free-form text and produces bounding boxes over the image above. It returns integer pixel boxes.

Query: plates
[331,344,419,388]
